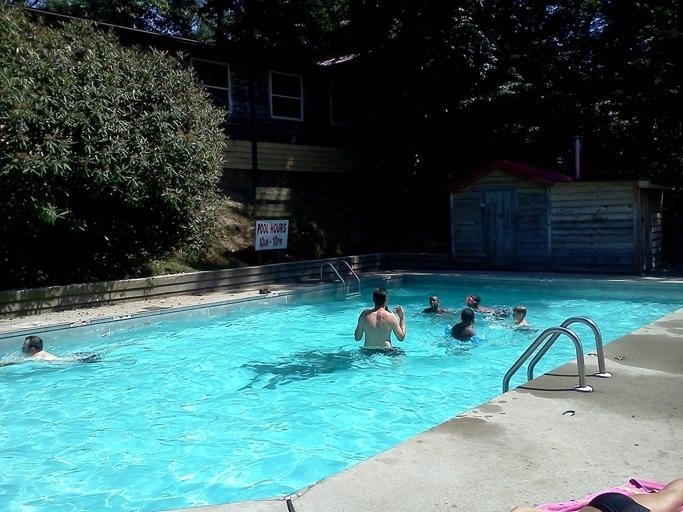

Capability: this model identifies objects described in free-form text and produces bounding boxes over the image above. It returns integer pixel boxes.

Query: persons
[451,307,475,340]
[377,287,404,345]
[509,478,683,512]
[451,294,512,320]
[0,336,100,369]
[355,288,406,348]
[505,305,529,329]
[421,295,449,316]
